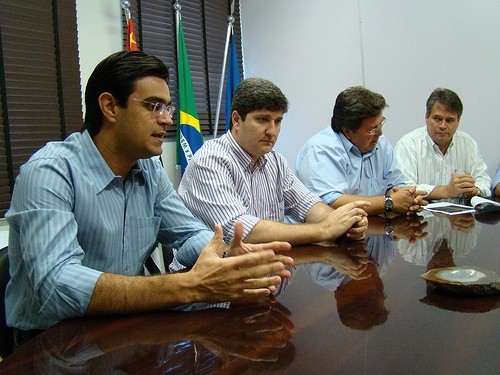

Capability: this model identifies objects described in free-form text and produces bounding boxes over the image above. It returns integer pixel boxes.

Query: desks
[0,196,500,375]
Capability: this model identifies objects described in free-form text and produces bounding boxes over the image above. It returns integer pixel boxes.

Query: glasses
[358,117,386,136]
[124,96,176,118]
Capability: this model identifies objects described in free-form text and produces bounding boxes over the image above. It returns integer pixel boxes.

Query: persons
[233,239,372,375]
[35,298,296,375]
[397,211,477,299]
[283,85,429,224]
[394,87,492,200]
[5,50,294,346]
[168,78,371,275]
[306,213,429,331]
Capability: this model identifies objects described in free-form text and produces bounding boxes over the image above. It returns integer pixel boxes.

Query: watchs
[384,218,395,238]
[384,194,394,213]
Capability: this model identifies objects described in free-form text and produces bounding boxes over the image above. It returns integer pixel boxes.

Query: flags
[125,19,140,51]
[225,34,240,132]
[176,13,204,179]
